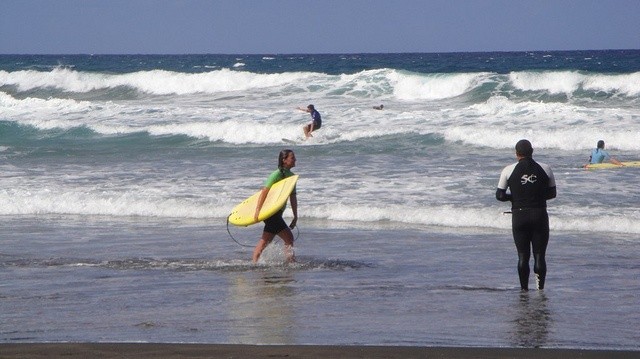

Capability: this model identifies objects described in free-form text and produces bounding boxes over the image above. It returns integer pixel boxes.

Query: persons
[296,104,321,141]
[495,139,558,299]
[588,140,625,168]
[251,149,301,265]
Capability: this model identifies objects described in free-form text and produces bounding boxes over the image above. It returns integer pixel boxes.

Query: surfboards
[228,175,298,226]
[580,161,640,167]
[282,138,308,144]
